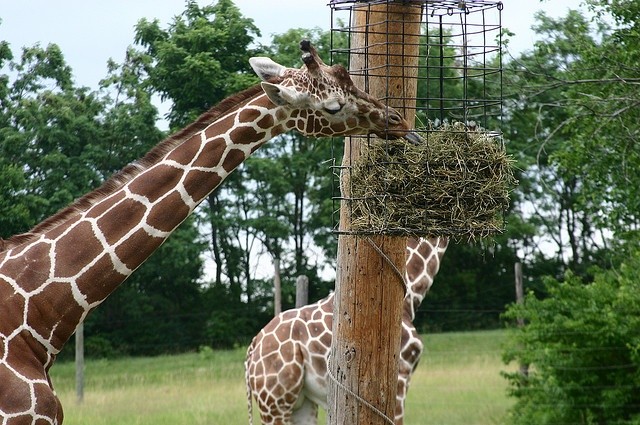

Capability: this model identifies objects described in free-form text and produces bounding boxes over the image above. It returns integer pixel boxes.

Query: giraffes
[0,40,409,425]
[244,119,477,425]
